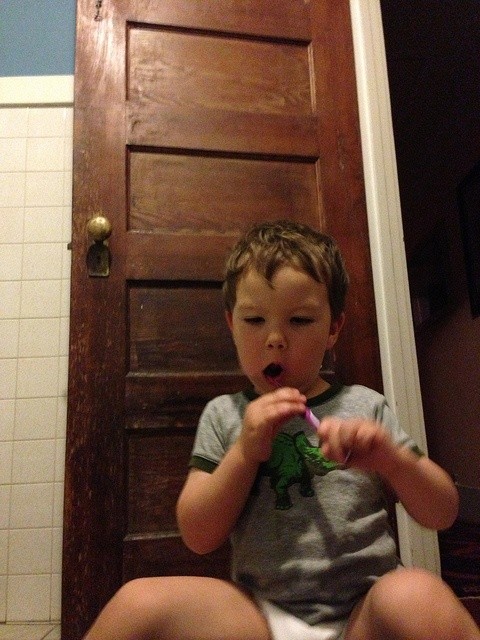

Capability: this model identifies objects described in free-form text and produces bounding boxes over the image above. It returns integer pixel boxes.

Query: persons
[79,220,479,640]
